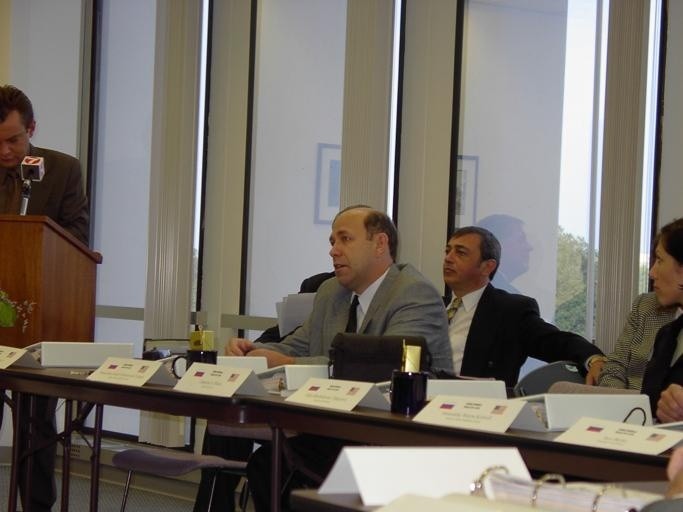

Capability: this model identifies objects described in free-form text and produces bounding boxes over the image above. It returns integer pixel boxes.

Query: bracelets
[589,356,607,367]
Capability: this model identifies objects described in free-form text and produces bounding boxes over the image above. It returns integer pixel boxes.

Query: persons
[598,218,683,389]
[641,314,683,423]
[442,227,607,388]
[195,205,453,512]
[0,85,89,247]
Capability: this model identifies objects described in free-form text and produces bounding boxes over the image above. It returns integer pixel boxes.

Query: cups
[172,350,218,380]
[390,372,428,415]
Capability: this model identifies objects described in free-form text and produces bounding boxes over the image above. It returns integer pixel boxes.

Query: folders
[21,341,134,368]
[256,365,334,393]
[508,393,654,432]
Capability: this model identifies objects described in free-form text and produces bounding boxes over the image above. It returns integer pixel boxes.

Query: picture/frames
[454,155,480,230]
[316,143,342,223]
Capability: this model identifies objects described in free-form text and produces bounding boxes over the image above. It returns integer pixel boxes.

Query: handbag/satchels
[328,333,431,384]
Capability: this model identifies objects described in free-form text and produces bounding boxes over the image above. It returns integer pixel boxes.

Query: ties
[343,294,360,334]
[447,298,461,321]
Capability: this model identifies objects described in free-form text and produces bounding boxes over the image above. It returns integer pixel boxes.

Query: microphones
[21,154,45,183]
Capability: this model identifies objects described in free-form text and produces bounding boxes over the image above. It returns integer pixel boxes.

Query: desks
[0,358,671,511]
